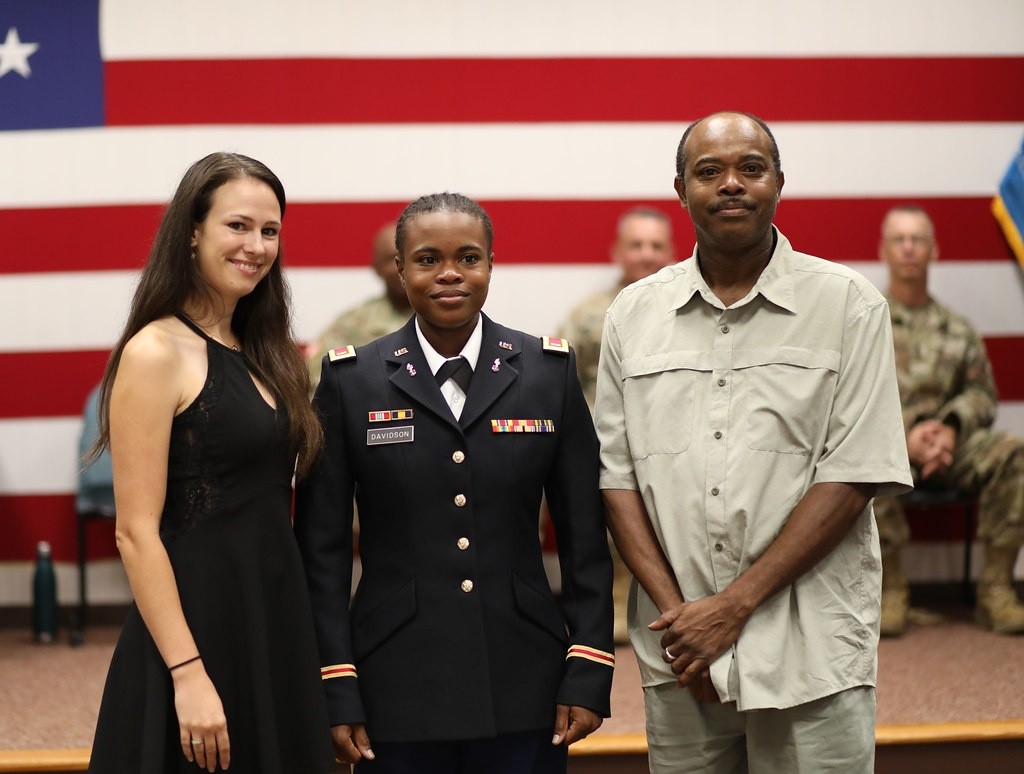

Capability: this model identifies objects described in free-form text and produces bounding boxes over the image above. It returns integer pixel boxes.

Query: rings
[192,740,203,745]
[666,647,676,662]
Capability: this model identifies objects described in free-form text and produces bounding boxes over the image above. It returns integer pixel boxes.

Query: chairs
[67,386,115,648]
[919,471,990,606]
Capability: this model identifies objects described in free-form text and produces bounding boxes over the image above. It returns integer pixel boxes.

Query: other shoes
[972,568,1024,634]
[877,578,909,636]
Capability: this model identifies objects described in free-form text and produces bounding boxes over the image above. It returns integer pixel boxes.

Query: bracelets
[167,654,202,672]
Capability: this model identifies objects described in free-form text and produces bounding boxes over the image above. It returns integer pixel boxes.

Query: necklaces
[206,330,238,351]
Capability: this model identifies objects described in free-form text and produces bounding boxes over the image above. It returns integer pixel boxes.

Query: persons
[593,111,915,774]
[304,204,1024,640]
[82,151,331,774]
[295,193,615,774]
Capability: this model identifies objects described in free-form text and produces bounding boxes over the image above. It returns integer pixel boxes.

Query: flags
[0,2,1024,611]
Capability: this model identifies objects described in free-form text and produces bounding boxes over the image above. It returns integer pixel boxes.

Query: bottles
[33,541,57,643]
